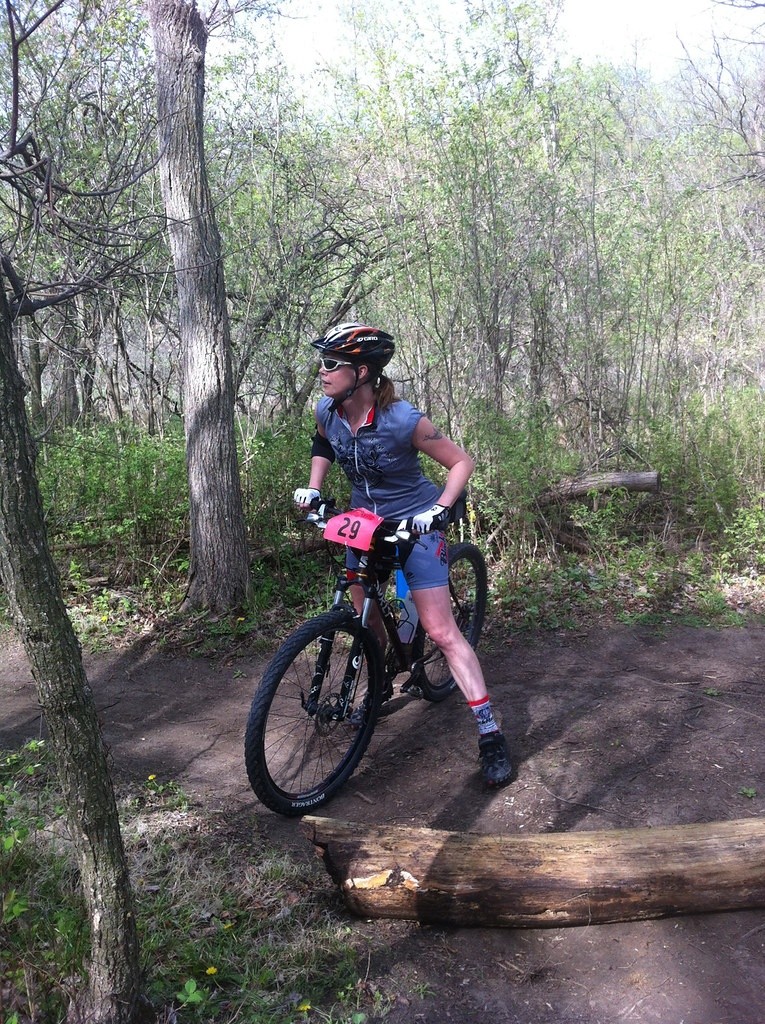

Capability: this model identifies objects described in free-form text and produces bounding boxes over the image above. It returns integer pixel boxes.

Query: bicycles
[244,499,487,816]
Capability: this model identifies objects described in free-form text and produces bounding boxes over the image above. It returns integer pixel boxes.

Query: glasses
[317,357,362,371]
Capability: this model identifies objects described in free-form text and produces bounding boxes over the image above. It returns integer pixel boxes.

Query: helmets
[311,321,396,369]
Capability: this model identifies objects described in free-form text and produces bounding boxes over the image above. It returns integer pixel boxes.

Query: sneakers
[350,681,394,731]
[476,735,510,785]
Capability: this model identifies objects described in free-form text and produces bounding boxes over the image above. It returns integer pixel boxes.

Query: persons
[294,322,513,786]
